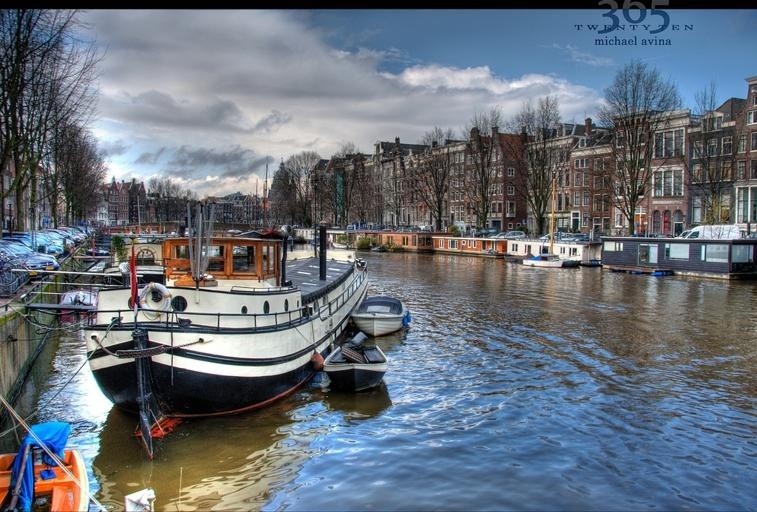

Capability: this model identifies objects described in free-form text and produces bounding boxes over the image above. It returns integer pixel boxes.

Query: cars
[398,223,434,234]
[539,231,590,242]
[0,224,98,280]
[475,228,529,239]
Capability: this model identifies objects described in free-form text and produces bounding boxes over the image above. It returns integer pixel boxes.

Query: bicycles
[0,254,32,298]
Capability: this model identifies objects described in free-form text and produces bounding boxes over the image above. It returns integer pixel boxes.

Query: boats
[521,253,582,268]
[0,448,91,512]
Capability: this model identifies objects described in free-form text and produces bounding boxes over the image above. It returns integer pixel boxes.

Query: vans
[677,224,741,239]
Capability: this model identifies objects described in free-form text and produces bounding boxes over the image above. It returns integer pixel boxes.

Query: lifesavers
[140,283,173,317]
